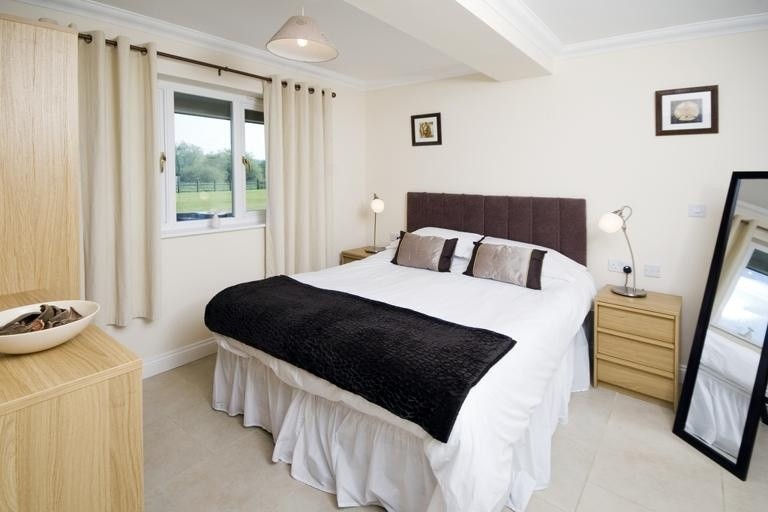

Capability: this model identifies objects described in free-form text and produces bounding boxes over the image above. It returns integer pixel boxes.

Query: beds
[685,329,761,460]
[204,192,599,512]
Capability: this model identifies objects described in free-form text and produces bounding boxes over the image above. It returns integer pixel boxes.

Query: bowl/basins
[0,300,101,356]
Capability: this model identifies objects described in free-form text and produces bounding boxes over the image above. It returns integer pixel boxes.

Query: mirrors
[671,170,768,481]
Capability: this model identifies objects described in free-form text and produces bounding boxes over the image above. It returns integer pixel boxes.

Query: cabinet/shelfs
[0,289,143,512]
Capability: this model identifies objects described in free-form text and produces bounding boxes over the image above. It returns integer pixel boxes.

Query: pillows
[387,226,585,290]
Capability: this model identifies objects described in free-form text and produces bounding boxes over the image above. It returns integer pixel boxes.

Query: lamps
[266,5,339,63]
[598,205,648,298]
[365,193,386,254]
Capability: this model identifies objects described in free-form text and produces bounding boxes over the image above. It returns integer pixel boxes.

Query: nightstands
[341,247,376,265]
[593,284,683,413]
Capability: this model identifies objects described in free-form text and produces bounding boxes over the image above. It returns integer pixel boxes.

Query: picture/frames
[655,85,719,136]
[410,112,442,146]
